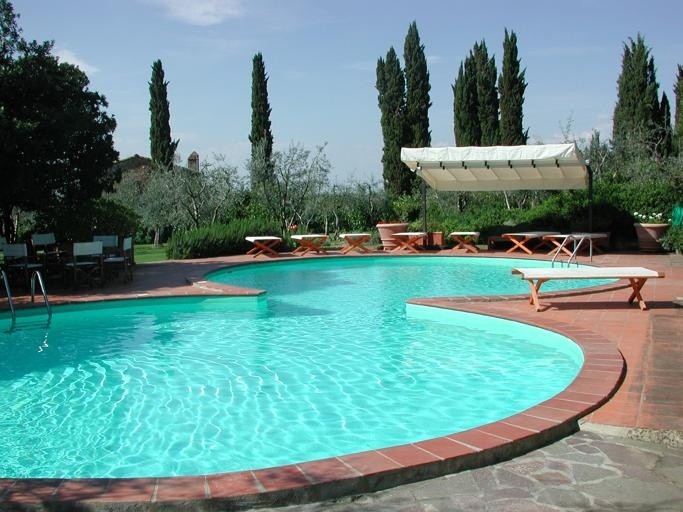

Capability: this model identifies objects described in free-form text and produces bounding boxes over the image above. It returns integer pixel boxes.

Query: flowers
[634,211,671,224]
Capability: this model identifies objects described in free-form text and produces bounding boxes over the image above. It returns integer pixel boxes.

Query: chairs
[2,233,134,297]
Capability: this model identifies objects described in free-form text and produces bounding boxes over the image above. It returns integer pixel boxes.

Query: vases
[633,223,669,249]
